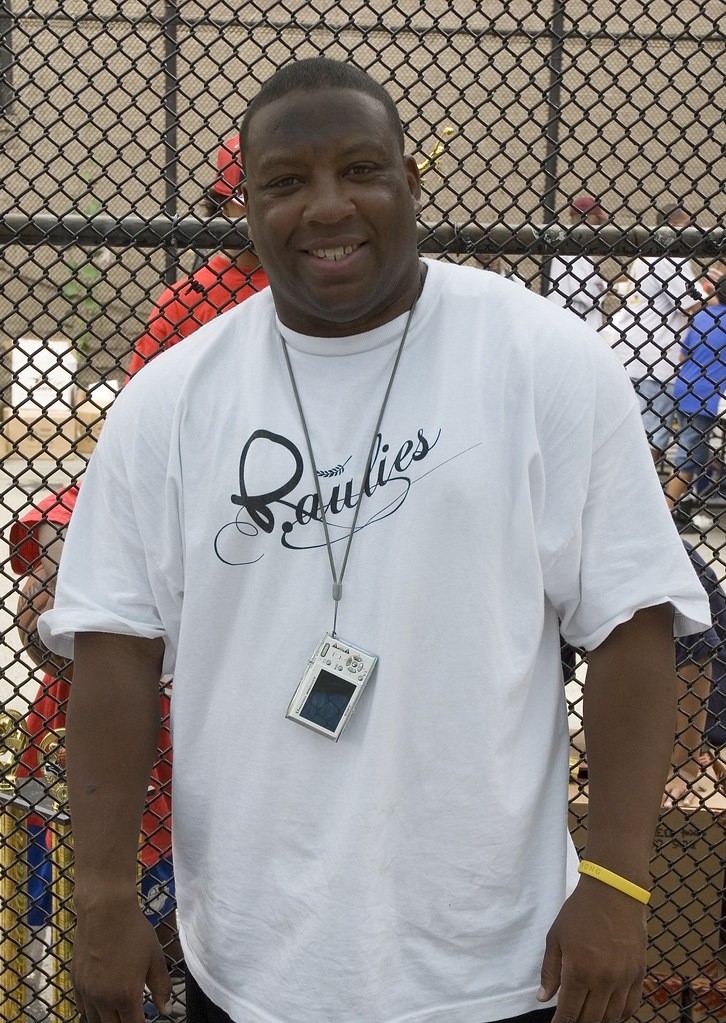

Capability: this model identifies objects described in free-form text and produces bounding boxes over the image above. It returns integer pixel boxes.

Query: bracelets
[577,860,652,905]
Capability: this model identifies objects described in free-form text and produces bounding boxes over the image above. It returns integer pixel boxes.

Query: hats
[213,139,251,211]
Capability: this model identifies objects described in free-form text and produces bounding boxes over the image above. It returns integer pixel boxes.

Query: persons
[9,474,185,1023]
[124,135,272,390]
[559,539,726,809]
[476,196,726,535]
[36,57,713,1023]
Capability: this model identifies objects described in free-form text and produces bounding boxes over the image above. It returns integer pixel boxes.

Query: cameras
[283,632,378,745]
